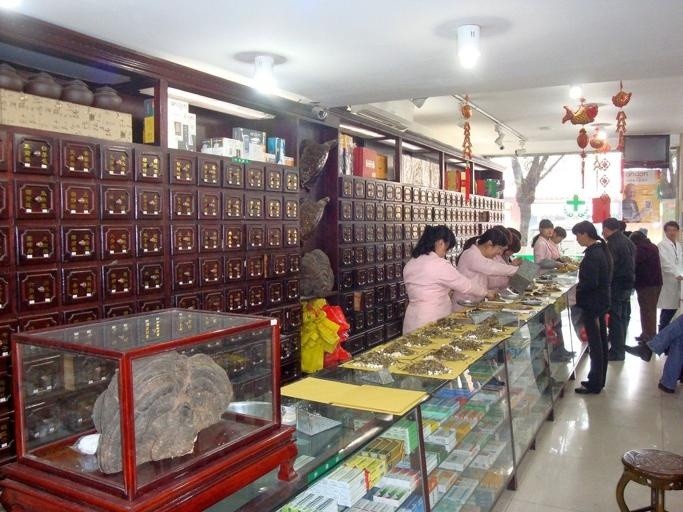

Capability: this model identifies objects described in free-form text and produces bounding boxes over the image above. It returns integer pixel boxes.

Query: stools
[613,447,682,511]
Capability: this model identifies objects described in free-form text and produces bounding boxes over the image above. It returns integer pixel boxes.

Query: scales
[456,299,479,318]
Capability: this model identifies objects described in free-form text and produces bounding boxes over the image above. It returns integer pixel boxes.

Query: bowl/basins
[227,400,287,421]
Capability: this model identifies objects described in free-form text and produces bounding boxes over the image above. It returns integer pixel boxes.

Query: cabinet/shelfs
[0,7,306,466]
[321,108,507,361]
[11,306,301,512]
[198,270,590,512]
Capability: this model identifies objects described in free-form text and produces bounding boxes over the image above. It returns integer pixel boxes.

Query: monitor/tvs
[622,134,670,168]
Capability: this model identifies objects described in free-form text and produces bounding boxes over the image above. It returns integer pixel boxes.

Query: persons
[657,221,683,332]
[623,313,683,393]
[548,226,567,262]
[528,306,576,396]
[622,182,644,223]
[571,219,615,394]
[628,230,663,346]
[450,225,522,312]
[402,224,497,336]
[600,216,637,361]
[530,219,565,270]
[617,219,632,323]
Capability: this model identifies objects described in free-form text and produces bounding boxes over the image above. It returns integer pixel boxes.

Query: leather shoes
[575,381,601,394]
[541,376,563,396]
[607,337,652,361]
[658,380,675,393]
[551,347,576,362]
